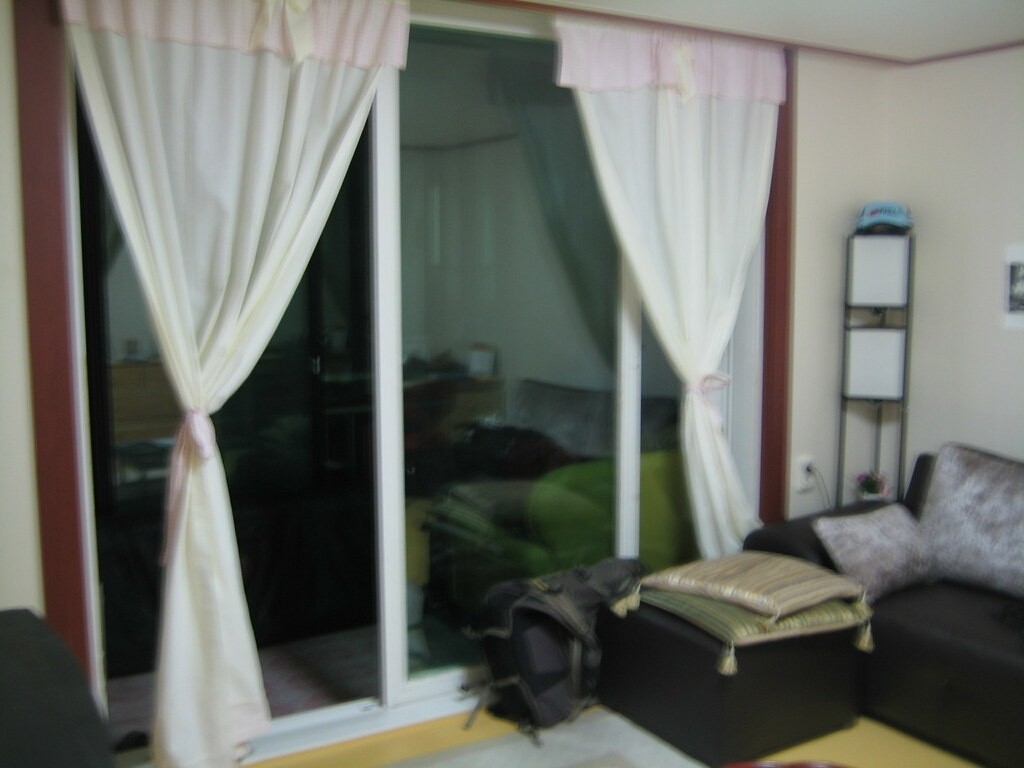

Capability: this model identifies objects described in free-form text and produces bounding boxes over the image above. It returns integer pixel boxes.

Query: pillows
[810,503,940,605]
[615,546,876,676]
[916,443,1024,597]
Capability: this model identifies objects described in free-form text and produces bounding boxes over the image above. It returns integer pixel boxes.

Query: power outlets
[793,455,820,492]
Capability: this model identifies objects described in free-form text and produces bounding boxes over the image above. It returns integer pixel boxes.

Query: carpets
[379,704,707,768]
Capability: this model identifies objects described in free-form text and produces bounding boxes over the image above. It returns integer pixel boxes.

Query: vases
[859,492,887,503]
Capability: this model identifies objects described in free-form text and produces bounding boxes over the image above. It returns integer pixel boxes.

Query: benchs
[110,361,190,445]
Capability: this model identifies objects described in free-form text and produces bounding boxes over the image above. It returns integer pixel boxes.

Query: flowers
[855,471,888,494]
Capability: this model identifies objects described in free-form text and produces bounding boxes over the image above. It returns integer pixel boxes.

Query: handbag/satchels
[457,420,557,484]
[464,556,643,749]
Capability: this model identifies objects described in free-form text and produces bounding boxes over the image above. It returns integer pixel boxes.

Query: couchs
[745,451,1024,768]
[421,380,695,643]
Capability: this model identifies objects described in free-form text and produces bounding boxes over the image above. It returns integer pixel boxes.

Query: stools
[593,603,861,768]
